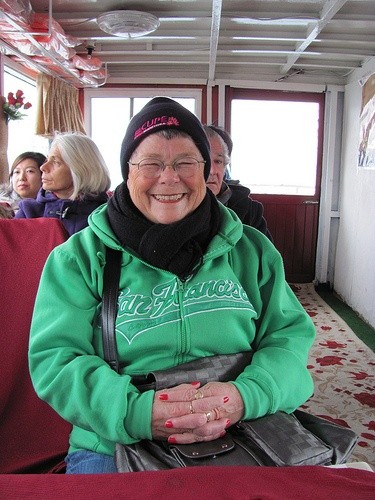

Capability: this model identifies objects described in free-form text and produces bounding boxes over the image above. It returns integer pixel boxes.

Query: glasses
[127,158,206,179]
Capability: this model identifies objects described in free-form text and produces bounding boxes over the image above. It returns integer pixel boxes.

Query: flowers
[1,89,32,126]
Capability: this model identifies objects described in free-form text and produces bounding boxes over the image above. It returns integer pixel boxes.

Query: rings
[213,407,220,420]
[195,390,202,399]
[205,411,210,422]
[190,401,193,414]
[194,434,205,441]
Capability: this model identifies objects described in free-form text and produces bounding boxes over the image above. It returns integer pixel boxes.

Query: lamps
[97,7,159,39]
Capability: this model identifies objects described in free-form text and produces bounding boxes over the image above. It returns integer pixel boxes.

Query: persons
[0,152,48,218]
[203,124,273,243]
[16,134,110,237]
[28,96,316,473]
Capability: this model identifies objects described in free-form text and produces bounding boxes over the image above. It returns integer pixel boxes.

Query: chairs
[0,214,82,473]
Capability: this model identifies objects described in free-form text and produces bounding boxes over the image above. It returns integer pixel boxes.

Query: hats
[120,96,211,182]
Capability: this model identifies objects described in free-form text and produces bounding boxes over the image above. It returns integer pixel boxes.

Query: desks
[0,460,375,500]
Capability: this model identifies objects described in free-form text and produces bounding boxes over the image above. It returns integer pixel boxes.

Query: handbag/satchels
[115,350,360,472]
